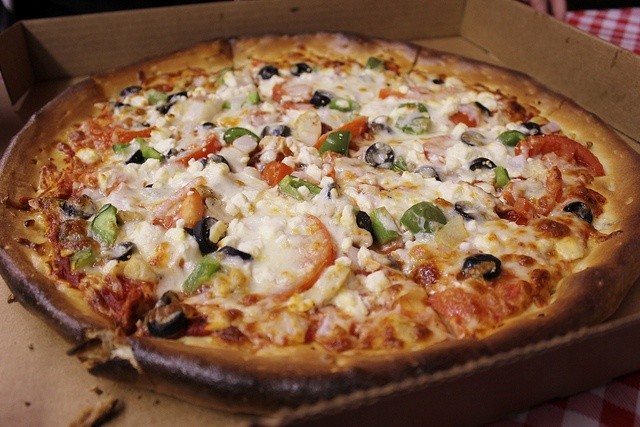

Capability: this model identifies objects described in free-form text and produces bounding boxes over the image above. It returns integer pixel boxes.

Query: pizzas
[0,30,640,414]
